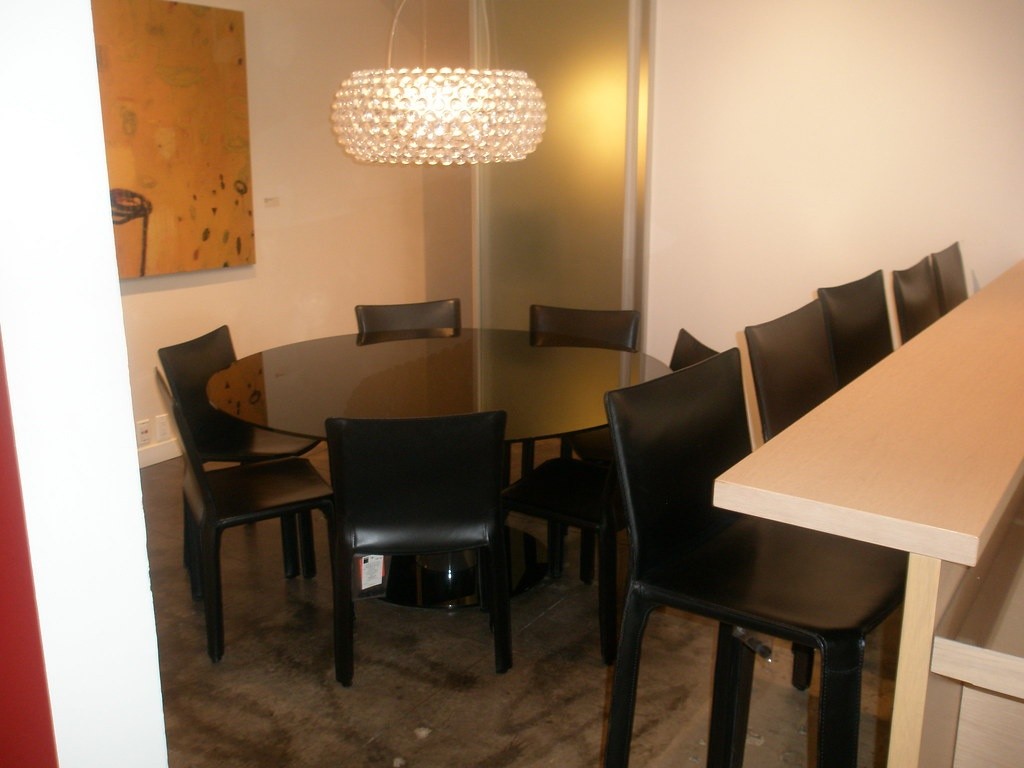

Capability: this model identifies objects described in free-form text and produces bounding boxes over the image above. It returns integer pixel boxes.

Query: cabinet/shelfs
[712,259,1024,768]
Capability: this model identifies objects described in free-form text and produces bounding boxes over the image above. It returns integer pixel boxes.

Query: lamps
[329,0,549,165]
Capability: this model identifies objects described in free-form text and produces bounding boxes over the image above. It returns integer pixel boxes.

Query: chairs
[154,243,972,768]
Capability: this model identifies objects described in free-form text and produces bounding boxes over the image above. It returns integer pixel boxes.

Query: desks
[204,328,675,611]
